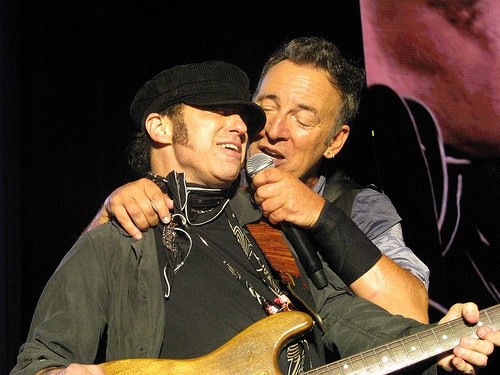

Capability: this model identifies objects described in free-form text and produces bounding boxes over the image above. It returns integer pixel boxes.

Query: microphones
[245,153,329,290]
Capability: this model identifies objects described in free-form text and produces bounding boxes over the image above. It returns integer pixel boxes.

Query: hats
[129,61,265,136]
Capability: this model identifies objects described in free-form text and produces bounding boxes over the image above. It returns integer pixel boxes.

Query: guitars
[94,299,500,374]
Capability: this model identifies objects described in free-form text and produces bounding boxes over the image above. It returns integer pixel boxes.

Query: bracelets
[304,197,383,286]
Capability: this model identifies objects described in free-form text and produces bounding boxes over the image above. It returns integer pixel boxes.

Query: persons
[7,60,500,375]
[78,35,431,328]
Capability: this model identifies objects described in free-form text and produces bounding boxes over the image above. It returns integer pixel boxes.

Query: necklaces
[178,199,313,375]
